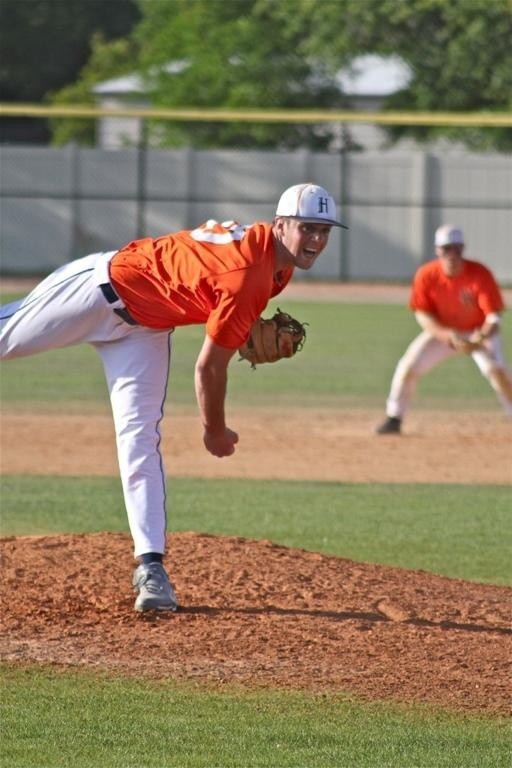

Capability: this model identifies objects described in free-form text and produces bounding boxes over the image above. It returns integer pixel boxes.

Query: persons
[376,222,512,435]
[0,183,350,612]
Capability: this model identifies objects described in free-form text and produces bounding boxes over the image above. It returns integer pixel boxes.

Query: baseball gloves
[238,307,309,371]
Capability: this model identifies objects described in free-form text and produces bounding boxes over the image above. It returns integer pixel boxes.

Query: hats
[434,224,464,247]
[275,184,350,230]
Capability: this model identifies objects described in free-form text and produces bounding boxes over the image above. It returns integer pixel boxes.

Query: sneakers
[376,418,400,433]
[133,561,178,614]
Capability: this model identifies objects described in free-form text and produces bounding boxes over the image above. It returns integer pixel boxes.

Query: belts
[99,282,138,327]
